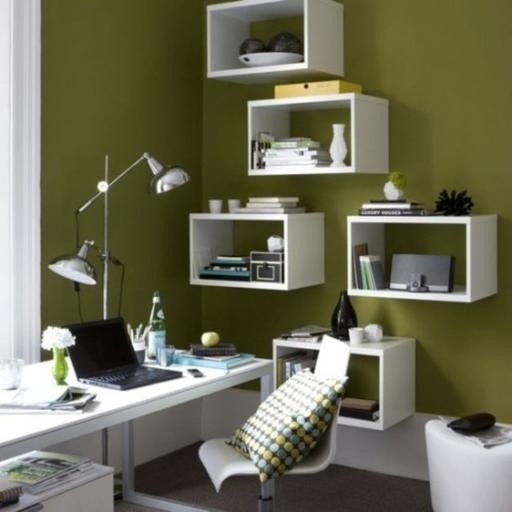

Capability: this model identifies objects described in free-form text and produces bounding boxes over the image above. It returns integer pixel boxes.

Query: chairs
[196,335,351,512]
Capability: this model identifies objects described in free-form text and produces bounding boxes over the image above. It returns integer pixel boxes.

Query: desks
[0,344,275,501]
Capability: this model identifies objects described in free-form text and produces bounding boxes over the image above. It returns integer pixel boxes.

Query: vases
[50,347,68,384]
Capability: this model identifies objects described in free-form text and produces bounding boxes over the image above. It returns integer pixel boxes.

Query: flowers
[40,325,77,351]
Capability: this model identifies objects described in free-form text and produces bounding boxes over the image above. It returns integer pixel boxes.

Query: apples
[201,331,220,347]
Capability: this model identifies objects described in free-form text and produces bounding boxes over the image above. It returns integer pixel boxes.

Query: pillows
[224,368,350,484]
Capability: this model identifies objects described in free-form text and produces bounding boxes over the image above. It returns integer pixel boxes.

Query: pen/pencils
[127,323,153,341]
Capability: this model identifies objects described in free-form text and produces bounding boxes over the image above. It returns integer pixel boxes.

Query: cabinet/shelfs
[201,0,346,80]
[271,317,417,432]
[0,450,116,512]
[341,212,501,310]
[189,207,326,297]
[241,95,393,180]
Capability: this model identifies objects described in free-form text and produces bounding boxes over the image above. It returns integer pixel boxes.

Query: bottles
[145,289,168,362]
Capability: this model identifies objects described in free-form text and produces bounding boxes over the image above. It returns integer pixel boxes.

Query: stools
[423,418,512,511]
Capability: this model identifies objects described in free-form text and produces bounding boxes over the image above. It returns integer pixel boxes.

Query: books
[352,243,385,289]
[358,199,432,216]
[232,197,306,214]
[339,398,379,422]
[276,350,317,390]
[251,131,332,169]
[0,450,95,496]
[173,343,256,370]
[0,383,97,414]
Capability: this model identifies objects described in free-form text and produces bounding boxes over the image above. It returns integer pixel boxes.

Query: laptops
[60,316,182,391]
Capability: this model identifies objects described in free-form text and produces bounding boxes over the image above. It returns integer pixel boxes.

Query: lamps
[47,151,191,322]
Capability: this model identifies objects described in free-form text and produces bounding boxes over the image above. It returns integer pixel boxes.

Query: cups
[2,357,26,390]
[363,322,384,343]
[349,326,363,344]
[154,343,175,366]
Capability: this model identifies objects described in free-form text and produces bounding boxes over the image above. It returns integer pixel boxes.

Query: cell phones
[187,369,203,377]
